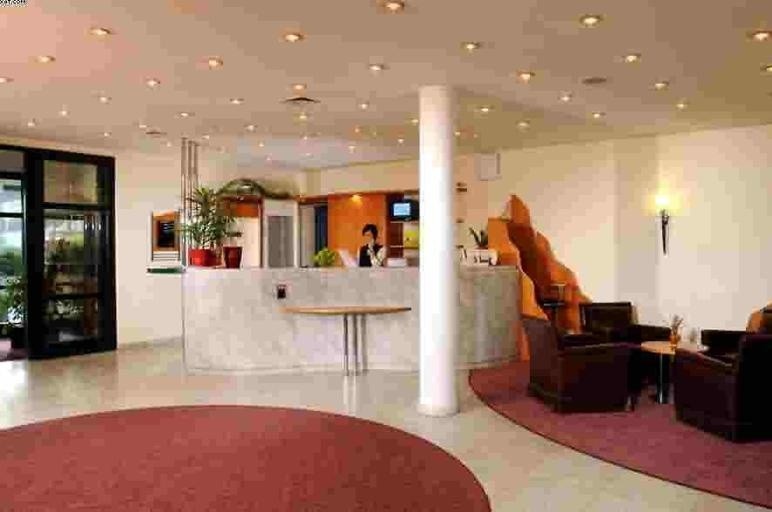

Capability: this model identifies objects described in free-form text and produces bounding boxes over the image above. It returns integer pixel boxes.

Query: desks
[285,307,413,377]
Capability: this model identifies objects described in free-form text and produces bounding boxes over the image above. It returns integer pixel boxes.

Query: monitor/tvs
[390,199,419,220]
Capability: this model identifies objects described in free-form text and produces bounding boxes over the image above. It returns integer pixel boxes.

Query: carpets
[0,404,490,512]
[469,361,772,509]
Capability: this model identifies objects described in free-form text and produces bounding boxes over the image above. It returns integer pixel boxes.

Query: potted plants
[309,246,340,267]
[468,227,488,249]
[172,186,249,268]
[4,252,25,349]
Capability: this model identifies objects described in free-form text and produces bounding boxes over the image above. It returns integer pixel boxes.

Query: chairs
[520,302,772,443]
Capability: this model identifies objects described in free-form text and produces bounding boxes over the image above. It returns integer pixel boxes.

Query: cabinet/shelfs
[327,191,419,267]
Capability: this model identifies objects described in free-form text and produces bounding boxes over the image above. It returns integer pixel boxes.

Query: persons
[356,223,385,267]
